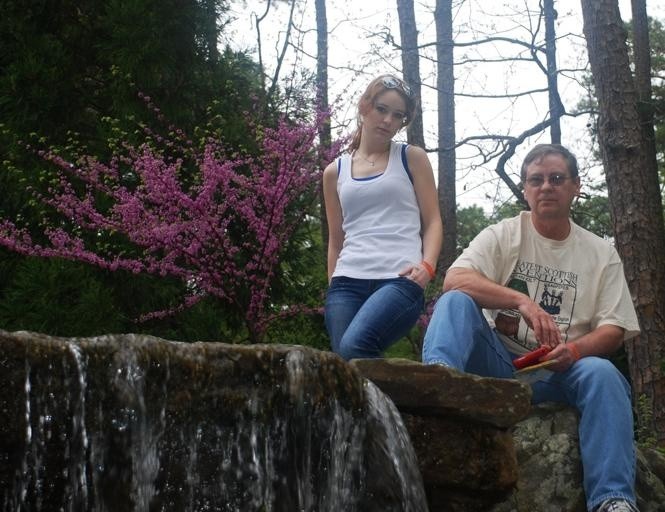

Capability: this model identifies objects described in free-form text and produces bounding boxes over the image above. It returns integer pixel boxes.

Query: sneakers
[597,498,639,512]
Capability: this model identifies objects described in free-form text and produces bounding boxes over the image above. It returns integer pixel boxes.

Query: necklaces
[357,150,386,168]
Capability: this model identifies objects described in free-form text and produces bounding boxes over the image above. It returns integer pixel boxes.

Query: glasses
[373,76,413,100]
[521,174,570,187]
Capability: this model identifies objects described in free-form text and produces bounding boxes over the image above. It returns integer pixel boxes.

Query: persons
[319,74,444,362]
[422,143,646,511]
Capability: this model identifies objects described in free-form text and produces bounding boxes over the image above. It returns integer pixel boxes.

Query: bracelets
[421,261,437,278]
[566,341,581,359]
